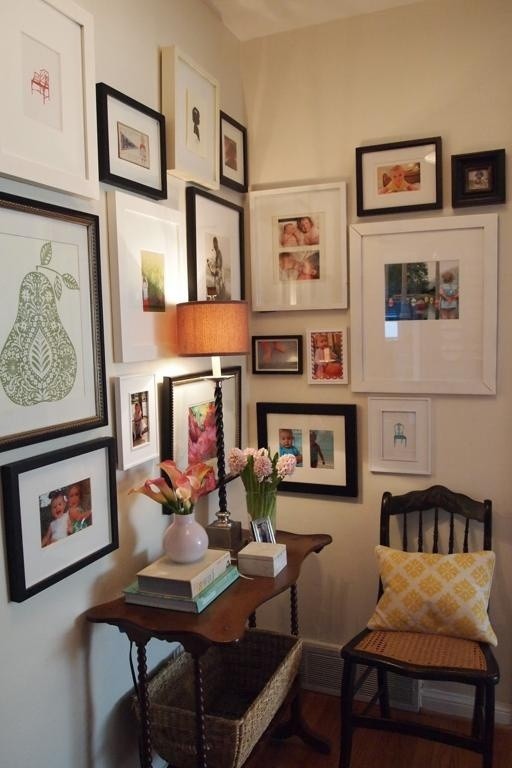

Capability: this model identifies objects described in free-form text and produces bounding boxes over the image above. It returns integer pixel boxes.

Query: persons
[279,430,304,468]
[132,403,147,439]
[205,237,228,301]
[379,164,416,191]
[42,491,74,547]
[278,224,301,249]
[297,218,320,244]
[65,486,92,531]
[298,250,321,280]
[435,270,458,319]
[309,430,328,470]
[311,334,335,378]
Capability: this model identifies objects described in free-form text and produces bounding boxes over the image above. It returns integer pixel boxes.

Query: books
[137,548,230,597]
[123,566,240,614]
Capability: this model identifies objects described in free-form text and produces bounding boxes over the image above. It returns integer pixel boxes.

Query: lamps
[177,301,250,565]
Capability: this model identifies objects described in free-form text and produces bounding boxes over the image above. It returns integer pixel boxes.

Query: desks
[84,528,332,768]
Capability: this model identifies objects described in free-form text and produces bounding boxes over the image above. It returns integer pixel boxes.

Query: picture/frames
[368,397,432,476]
[255,402,358,498]
[0,437,120,602]
[186,186,246,302]
[162,365,242,515]
[105,190,189,365]
[348,214,499,396]
[113,372,161,471]
[0,192,109,453]
[251,515,276,544]
[356,136,443,217]
[96,82,167,200]
[307,329,348,386]
[248,181,347,313]
[0,1,100,203]
[451,148,506,209]
[161,46,221,192]
[252,334,302,376]
[219,110,248,194]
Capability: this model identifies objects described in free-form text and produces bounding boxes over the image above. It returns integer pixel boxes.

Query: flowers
[129,459,217,515]
[229,447,296,516]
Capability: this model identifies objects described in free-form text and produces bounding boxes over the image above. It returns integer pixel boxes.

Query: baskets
[131,627,304,768]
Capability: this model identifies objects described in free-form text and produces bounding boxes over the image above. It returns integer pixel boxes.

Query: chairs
[337,485,500,768]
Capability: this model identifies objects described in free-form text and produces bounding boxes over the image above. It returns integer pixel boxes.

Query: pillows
[366,545,498,648]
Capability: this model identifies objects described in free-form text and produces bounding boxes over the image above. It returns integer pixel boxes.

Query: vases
[244,489,279,541]
[164,510,209,565]
